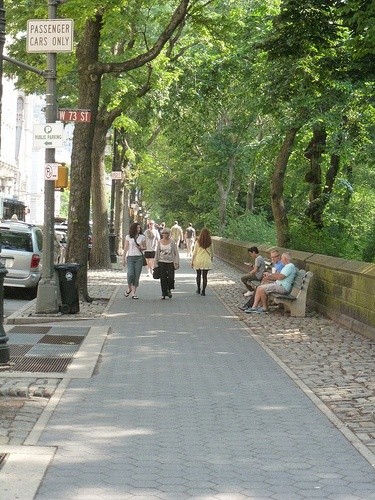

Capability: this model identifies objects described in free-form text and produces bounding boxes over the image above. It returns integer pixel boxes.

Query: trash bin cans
[55,262,80,314]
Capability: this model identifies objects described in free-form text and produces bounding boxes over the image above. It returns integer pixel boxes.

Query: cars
[1,214,93,265]
[0,221,44,289]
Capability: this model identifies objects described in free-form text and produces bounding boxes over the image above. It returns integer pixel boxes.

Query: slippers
[132,295,139,299]
[124,289,132,297]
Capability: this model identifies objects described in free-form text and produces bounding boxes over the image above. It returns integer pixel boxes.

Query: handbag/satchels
[153,267,160,279]
[142,258,147,266]
[144,251,155,257]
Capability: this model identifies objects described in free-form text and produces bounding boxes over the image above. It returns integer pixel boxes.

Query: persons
[156,228,179,299]
[245,252,296,313]
[241,247,266,297]
[144,219,160,277]
[122,223,147,299]
[171,221,183,250]
[158,222,165,239]
[240,250,284,311]
[192,230,214,296]
[184,223,196,257]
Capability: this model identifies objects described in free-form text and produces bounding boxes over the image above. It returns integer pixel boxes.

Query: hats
[149,220,154,223]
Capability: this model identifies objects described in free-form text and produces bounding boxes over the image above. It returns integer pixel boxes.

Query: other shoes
[201,293,205,296]
[169,292,172,298]
[244,291,254,296]
[256,308,269,313]
[238,305,252,311]
[245,308,258,312]
[161,297,165,300]
[196,290,200,294]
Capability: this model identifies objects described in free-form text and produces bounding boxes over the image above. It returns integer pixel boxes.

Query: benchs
[247,260,313,319]
